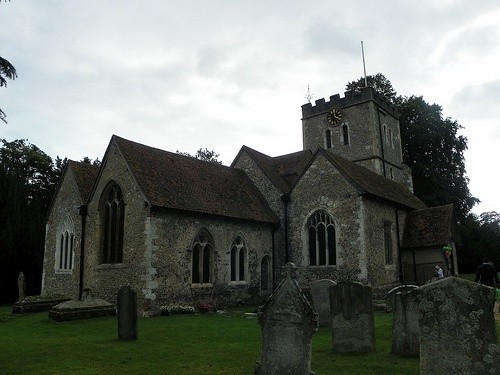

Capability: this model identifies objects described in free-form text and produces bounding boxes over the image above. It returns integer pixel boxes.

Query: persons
[434,266,444,277]
[474,257,500,314]
[488,258,497,289]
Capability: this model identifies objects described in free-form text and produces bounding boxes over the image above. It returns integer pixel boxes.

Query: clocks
[326,107,344,127]
